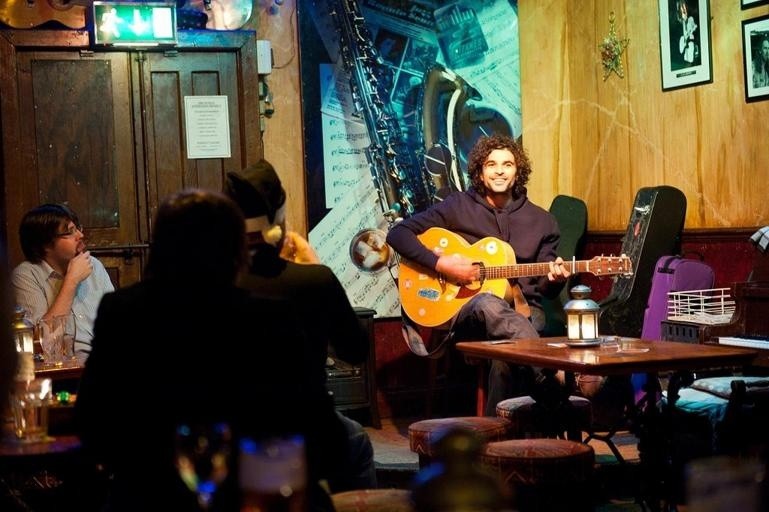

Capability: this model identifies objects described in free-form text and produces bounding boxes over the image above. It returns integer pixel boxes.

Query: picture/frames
[742,14,769,104]
[740,0,769,11]
[658,0,713,93]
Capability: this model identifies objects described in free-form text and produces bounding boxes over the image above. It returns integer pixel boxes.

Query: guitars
[396,226,633,329]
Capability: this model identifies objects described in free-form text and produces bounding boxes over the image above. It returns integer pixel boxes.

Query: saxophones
[330,1,482,229]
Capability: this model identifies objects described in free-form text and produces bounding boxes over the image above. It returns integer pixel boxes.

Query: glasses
[54,224,82,236]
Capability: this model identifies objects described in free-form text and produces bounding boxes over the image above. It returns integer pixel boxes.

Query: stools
[422,325,478,418]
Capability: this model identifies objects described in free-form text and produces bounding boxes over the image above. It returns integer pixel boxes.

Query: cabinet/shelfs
[325,304,383,429]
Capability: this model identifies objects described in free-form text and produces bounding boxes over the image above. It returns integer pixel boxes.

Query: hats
[223,159,286,245]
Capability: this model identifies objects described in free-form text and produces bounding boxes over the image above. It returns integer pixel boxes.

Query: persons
[74,183,376,512]
[752,33,769,87]
[217,158,373,495]
[386,130,608,423]
[1,202,119,363]
[673,0,700,67]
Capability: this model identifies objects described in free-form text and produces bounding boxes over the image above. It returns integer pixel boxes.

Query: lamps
[563,284,603,348]
[8,305,36,359]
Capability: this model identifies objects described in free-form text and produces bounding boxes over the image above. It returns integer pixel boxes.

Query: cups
[56,312,78,362]
[603,344,619,356]
[7,386,49,441]
[38,316,64,368]
